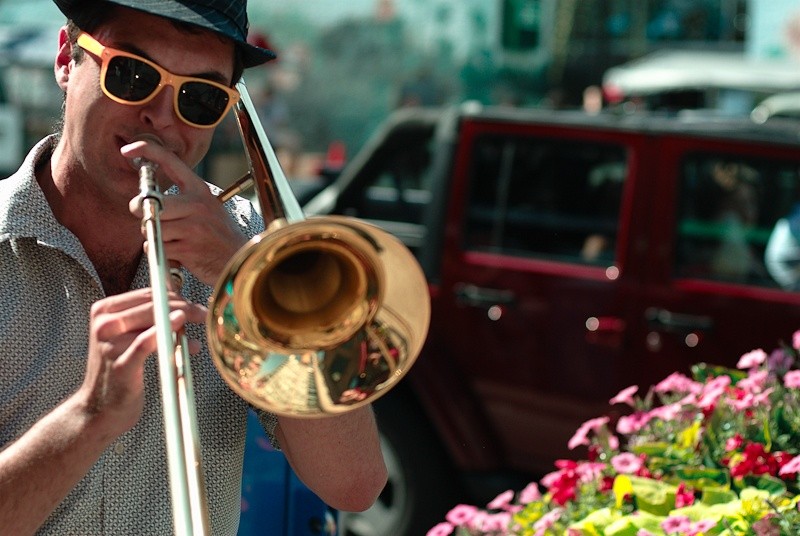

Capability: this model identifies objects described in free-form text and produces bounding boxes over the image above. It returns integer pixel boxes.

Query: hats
[53,0,277,68]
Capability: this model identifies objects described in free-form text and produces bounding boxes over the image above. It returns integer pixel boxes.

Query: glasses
[69,26,240,129]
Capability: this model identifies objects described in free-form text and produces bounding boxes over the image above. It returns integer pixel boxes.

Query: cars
[299,36,800,535]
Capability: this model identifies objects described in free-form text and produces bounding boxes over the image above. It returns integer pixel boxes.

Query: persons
[0,1,387,535]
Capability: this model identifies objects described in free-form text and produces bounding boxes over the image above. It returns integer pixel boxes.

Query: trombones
[123,74,432,536]
